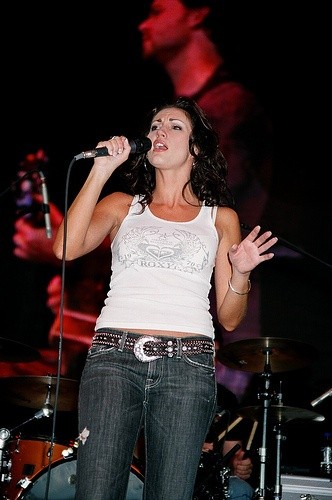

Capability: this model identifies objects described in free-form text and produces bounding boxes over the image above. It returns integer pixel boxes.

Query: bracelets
[228,277,251,295]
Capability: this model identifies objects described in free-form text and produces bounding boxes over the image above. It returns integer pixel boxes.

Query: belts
[91,331,214,363]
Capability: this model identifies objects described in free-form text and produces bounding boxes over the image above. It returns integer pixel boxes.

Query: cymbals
[0,376,80,413]
[218,336,300,374]
[238,404,325,424]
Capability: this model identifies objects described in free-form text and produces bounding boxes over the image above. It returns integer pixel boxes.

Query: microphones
[75,137,153,160]
[39,171,52,238]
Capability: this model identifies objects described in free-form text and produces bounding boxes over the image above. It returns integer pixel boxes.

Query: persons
[53,95,278,500]
[194,318,257,500]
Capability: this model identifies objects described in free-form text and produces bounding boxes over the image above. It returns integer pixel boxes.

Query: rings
[112,136,116,138]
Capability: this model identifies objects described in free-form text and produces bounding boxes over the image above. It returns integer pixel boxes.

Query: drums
[3,433,79,500]
[13,453,145,500]
